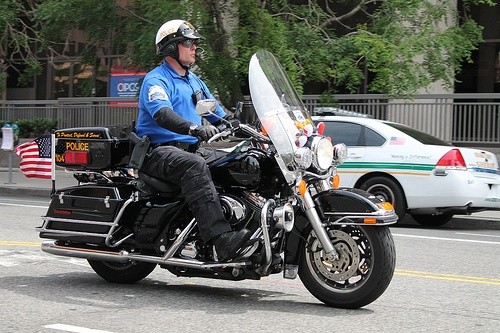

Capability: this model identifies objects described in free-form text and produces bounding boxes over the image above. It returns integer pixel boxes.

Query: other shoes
[214,229,251,263]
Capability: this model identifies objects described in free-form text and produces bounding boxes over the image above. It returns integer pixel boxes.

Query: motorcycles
[35,48,399,309]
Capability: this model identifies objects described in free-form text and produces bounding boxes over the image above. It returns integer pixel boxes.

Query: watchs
[189,124,198,136]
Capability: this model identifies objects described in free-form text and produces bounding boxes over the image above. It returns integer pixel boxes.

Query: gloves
[182,121,220,143]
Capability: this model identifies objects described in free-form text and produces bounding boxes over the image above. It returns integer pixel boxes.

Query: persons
[136,19,262,262]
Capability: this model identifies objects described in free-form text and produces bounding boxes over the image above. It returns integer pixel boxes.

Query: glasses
[178,40,199,48]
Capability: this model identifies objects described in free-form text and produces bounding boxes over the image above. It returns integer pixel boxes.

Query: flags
[15,134,52,180]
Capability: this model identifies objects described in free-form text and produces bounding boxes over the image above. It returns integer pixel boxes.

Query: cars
[309,115,500,228]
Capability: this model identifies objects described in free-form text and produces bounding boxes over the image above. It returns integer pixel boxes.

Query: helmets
[154,19,203,55]
